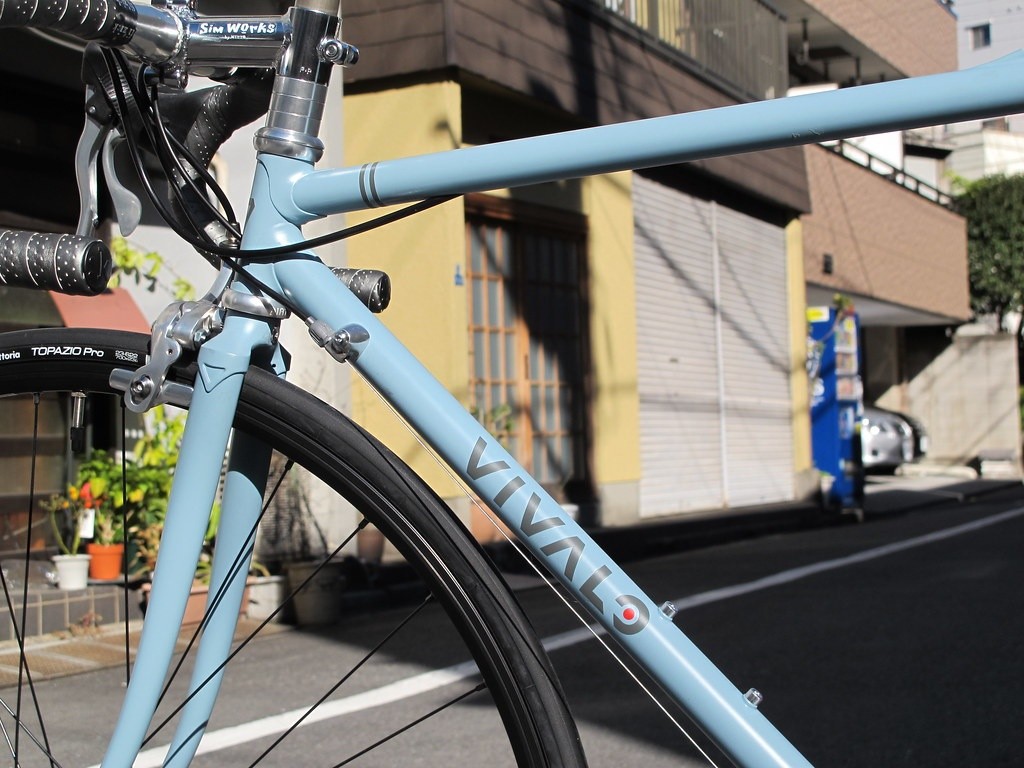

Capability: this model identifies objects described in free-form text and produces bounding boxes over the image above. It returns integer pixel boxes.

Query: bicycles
[0,3,1023,767]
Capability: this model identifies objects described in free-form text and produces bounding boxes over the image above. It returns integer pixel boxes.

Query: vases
[358,530,385,559]
[284,554,349,629]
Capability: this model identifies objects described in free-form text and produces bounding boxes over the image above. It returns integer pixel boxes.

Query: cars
[859,403,929,472]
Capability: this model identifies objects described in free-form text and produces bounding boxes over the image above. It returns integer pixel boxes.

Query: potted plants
[37,396,286,629]
